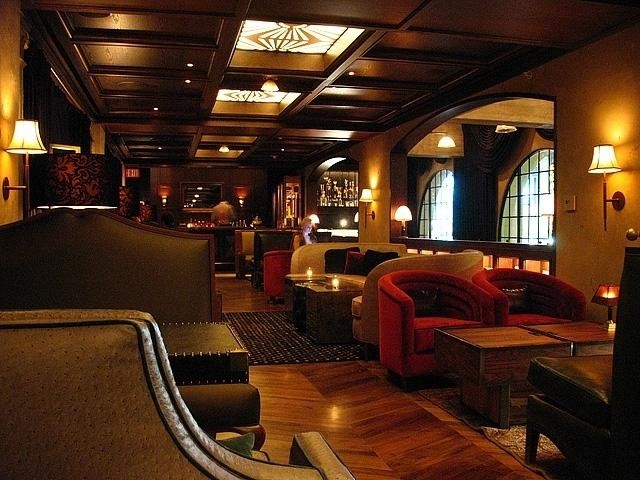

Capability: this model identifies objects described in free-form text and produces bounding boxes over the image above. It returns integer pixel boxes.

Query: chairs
[263,250,295,304]
[472,268,586,327]
[378,270,495,391]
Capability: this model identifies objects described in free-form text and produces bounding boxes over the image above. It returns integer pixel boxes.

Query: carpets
[222,311,372,365]
[417,387,595,480]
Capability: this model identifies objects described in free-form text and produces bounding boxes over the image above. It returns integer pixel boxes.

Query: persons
[210,195,237,226]
[292,217,318,250]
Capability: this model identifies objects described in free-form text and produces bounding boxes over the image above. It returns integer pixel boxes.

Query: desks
[157,323,249,385]
[285,274,362,344]
[433,321,615,429]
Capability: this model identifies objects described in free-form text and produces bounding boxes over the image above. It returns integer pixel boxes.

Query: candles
[307,266,312,281]
[332,275,340,291]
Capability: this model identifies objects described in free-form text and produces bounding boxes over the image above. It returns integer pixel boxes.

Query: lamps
[591,282,620,332]
[3,119,47,216]
[358,189,375,219]
[260,80,279,93]
[586,143,626,231]
[394,206,412,239]
[430,132,456,149]
[495,125,517,133]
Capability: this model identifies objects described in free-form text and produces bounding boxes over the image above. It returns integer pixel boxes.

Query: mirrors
[179,181,224,213]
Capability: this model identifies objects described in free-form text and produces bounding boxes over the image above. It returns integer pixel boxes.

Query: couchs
[0,210,266,448]
[249,231,331,288]
[352,248,483,346]
[0,309,357,479]
[525,246,640,480]
[283,243,406,325]
[235,230,254,279]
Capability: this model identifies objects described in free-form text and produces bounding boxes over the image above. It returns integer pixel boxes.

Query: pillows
[326,245,398,275]
